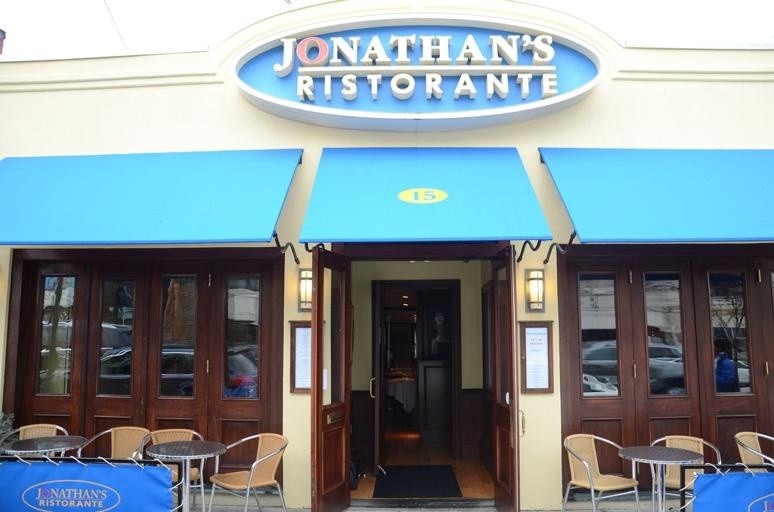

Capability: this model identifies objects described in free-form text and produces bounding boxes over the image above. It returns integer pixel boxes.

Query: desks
[618,445,704,512]
[2,434,87,462]
[146,440,227,512]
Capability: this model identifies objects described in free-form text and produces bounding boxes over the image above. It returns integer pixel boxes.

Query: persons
[431,312,448,355]
[715,346,738,392]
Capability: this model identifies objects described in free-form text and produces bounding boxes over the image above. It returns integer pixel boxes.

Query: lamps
[296,267,313,311]
[526,269,544,311]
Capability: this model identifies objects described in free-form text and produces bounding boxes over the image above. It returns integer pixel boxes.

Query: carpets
[373,464,465,497]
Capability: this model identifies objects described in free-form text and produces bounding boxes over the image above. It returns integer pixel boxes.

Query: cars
[580,340,749,395]
[40,321,259,396]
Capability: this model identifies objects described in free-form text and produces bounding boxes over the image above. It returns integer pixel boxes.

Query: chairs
[141,428,206,512]
[208,432,288,512]
[0,423,70,463]
[563,433,640,512]
[77,426,152,464]
[734,431,774,473]
[650,435,722,512]
